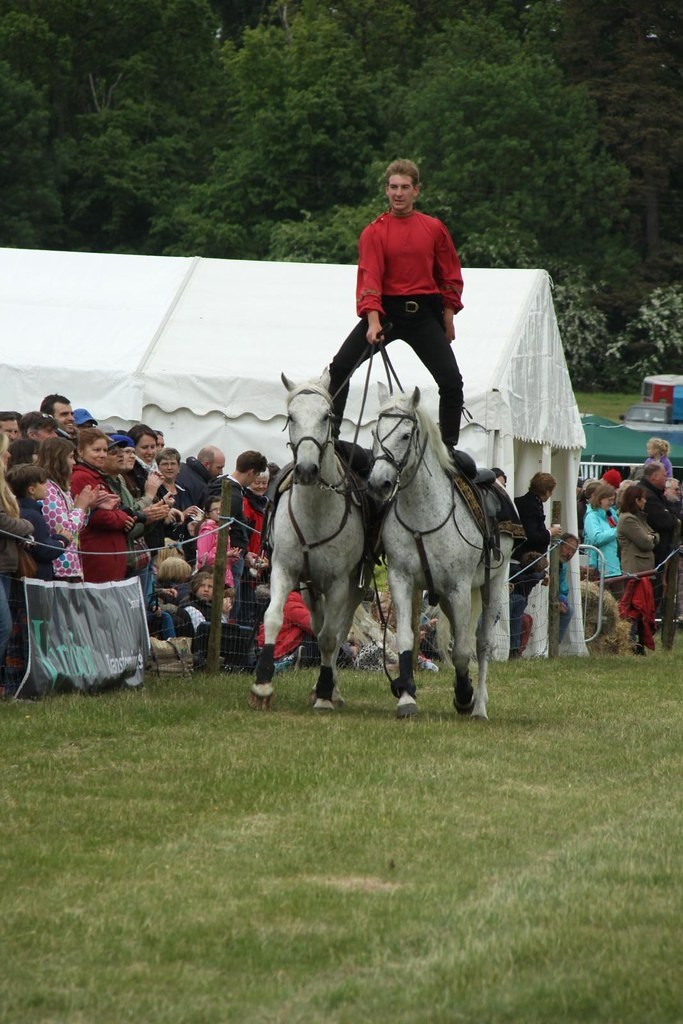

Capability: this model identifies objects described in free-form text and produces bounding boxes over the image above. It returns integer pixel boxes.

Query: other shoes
[510,650,520,659]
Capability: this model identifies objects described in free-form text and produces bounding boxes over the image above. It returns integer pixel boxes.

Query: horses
[363,380,521,722]
[248,363,391,715]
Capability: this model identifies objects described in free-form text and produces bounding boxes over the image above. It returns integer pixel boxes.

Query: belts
[393,301,433,313]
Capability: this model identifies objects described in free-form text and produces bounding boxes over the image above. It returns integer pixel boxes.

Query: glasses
[208,508,221,514]
[110,448,124,454]
[154,431,163,436]
[42,413,53,418]
[666,488,679,491]
[125,449,136,455]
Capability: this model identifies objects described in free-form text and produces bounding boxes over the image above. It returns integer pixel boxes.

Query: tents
[0,247,590,658]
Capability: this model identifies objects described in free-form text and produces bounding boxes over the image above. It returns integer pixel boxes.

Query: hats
[603,469,621,488]
[111,435,134,446]
[577,478,586,487]
[73,409,97,425]
[106,435,128,448]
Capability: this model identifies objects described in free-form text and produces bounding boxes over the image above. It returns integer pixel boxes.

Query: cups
[552,524,560,538]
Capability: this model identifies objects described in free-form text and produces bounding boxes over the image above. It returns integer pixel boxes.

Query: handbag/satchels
[150,637,194,682]
[19,547,39,578]
[580,566,599,581]
[191,622,255,674]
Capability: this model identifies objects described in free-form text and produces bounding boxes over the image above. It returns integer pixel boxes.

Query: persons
[0,394,683,688]
[324,158,465,460]
